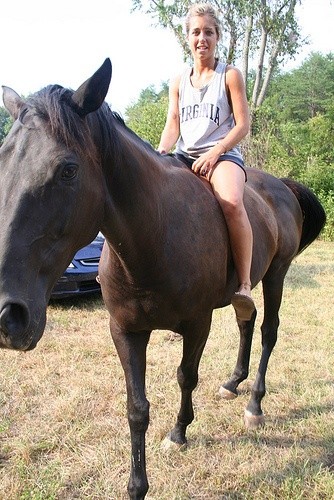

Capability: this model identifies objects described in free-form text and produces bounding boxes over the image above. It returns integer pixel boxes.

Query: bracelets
[214,141,229,157]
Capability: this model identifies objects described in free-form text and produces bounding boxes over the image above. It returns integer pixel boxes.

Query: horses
[1,56,327,500]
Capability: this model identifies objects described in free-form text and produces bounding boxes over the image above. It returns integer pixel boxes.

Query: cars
[50,231,106,299]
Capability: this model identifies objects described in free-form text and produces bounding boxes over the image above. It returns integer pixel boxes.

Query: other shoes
[232,282,254,323]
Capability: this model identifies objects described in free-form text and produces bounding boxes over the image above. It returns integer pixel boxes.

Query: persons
[152,5,258,329]
